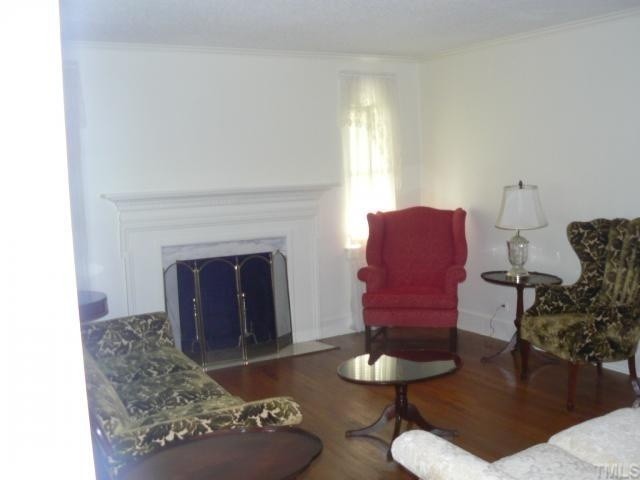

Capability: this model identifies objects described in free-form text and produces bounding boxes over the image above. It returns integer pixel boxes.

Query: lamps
[496,181,549,280]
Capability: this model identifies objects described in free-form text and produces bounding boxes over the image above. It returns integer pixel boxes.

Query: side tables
[78,292,107,322]
[480,271,562,382]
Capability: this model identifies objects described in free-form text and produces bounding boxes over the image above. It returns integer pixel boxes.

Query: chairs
[357,206,467,351]
[520,218,640,409]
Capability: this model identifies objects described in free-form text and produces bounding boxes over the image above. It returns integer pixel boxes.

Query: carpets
[200,340,340,371]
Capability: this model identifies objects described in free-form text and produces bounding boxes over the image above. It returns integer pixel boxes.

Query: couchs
[81,313,321,480]
[391,402,640,480]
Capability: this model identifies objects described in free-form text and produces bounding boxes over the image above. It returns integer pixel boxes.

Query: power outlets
[500,304,507,309]
